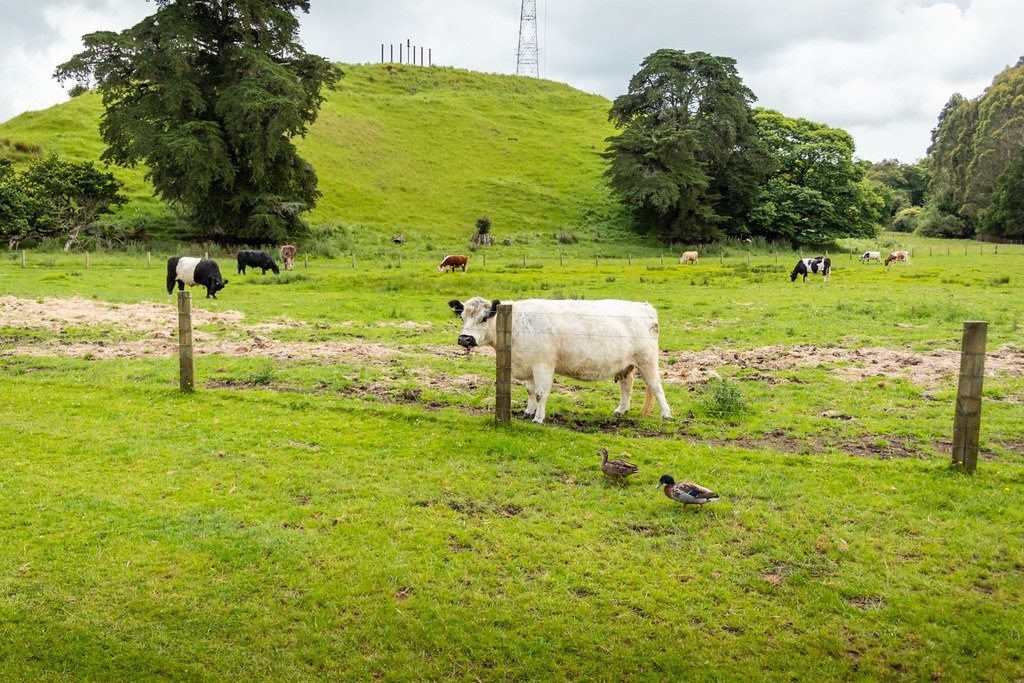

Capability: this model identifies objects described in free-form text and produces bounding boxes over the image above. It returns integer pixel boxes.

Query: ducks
[655,475,720,514]
[595,447,643,487]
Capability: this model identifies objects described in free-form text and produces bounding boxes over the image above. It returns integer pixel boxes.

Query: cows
[858,251,882,265]
[437,254,468,273]
[448,296,676,422]
[679,251,698,265]
[167,245,296,298]
[885,251,911,267]
[790,257,831,283]
